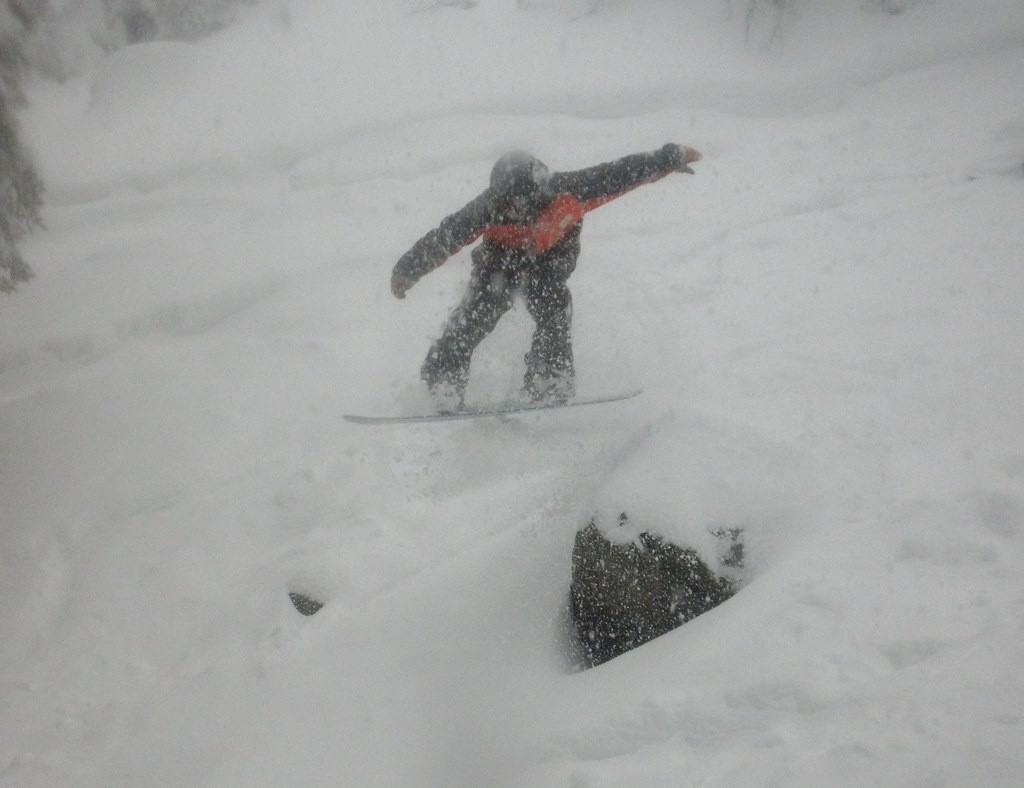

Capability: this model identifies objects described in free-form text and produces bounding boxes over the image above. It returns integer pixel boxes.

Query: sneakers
[428,374,469,414]
[524,371,577,404]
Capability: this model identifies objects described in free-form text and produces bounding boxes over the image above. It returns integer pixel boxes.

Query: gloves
[390,274,417,299]
[683,146,702,174]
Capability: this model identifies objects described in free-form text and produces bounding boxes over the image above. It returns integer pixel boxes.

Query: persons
[390,141,705,418]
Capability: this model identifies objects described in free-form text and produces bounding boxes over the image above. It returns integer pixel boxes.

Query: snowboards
[342,388,645,425]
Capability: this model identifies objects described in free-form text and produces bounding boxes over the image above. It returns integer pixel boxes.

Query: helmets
[490,151,557,200]
